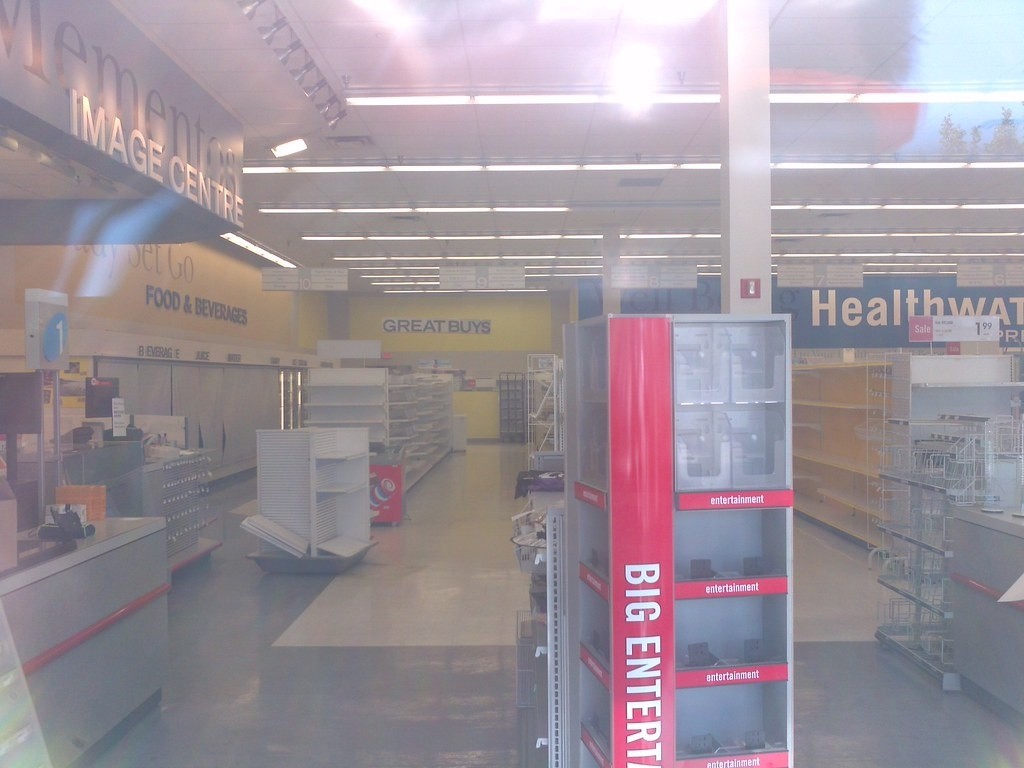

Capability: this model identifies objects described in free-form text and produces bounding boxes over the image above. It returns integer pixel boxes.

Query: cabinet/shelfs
[498,312,795,768]
[237,367,454,574]
[792,354,1017,559]
